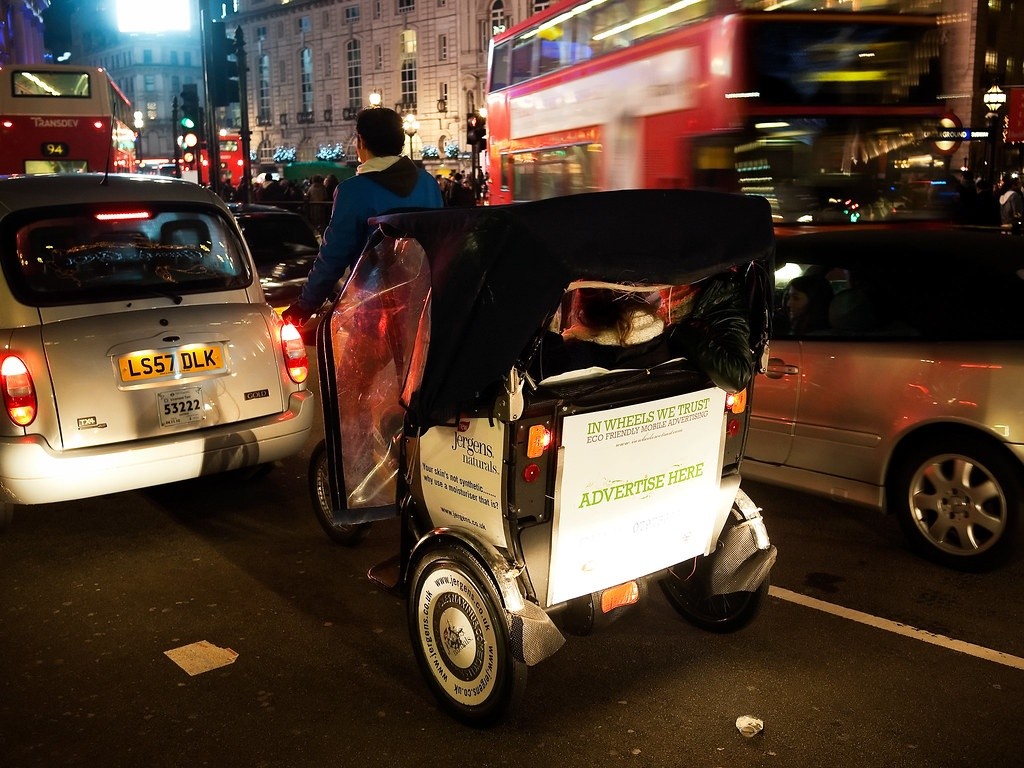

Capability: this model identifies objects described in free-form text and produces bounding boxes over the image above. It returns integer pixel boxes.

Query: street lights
[403,114,419,161]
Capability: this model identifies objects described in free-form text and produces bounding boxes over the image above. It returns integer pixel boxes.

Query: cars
[740,216,1024,575]
[0,171,314,526]
[223,199,347,302]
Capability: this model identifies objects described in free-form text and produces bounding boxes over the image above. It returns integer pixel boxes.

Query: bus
[483,0,1008,237]
[198,131,249,185]
[0,65,139,174]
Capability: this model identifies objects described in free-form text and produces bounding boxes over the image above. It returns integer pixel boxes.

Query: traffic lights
[481,117,488,148]
[212,18,250,102]
[180,83,200,148]
[467,110,481,144]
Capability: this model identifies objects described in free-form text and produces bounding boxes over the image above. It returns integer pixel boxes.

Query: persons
[436,169,489,208]
[199,173,338,204]
[281,108,444,474]
[845,157,901,221]
[940,169,1024,227]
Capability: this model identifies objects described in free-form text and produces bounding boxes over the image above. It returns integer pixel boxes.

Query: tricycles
[280,189,781,733]
